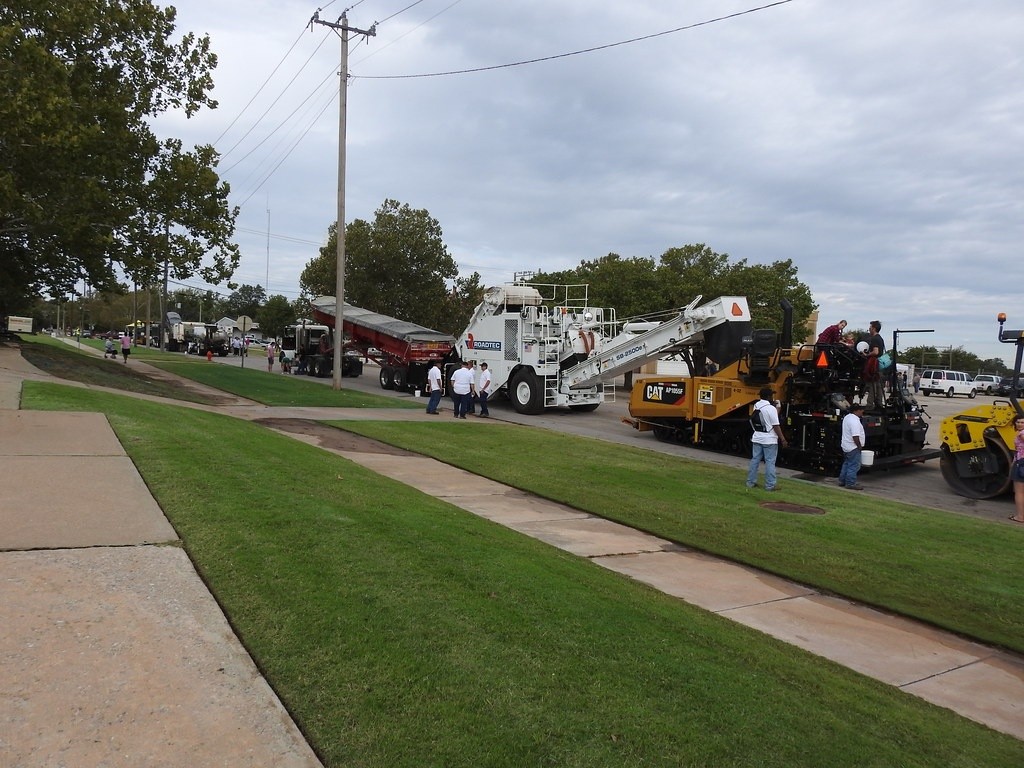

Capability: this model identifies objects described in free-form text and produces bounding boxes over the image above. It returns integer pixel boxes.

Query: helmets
[856,341,869,353]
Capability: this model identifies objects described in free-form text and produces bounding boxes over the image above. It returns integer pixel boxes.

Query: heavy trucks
[176,321,228,357]
[279,295,457,391]
[168,323,178,350]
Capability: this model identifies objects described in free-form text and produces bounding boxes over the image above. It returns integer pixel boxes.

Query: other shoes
[753,483,760,487]
[771,486,781,491]
[454,415,458,418]
[459,416,466,419]
[482,413,490,418]
[426,411,439,414]
[839,481,846,486]
[465,411,476,414]
[480,411,484,415]
[846,484,863,490]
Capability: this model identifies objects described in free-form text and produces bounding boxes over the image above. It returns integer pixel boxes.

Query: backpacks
[749,404,774,432]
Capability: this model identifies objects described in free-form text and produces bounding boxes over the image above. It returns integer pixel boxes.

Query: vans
[973,375,1003,396]
[919,369,978,398]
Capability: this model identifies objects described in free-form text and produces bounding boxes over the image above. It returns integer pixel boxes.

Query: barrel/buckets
[861,450,874,465]
[415,390,421,397]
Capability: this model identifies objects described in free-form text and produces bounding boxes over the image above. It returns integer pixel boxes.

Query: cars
[257,339,270,344]
[265,341,275,348]
[83,331,91,336]
[345,351,358,356]
[249,339,266,348]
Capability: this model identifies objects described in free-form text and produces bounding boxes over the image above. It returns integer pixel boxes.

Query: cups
[835,409,840,415]
[471,395,474,397]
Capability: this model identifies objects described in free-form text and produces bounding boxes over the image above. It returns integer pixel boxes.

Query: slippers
[1009,515,1023,523]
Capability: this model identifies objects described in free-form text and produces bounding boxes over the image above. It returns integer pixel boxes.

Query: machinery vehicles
[621,297,940,475]
[438,282,752,415]
[940,313,1024,499]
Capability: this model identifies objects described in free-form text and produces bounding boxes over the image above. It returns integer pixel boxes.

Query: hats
[462,362,469,366]
[479,362,487,366]
[849,403,864,412]
[757,387,777,395]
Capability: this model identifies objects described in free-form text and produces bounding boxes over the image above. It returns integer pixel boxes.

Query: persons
[479,362,491,417]
[838,403,865,490]
[450,361,475,419]
[746,387,789,491]
[268,338,281,370]
[813,320,855,377]
[76,326,79,341]
[106,332,132,363]
[896,371,921,394]
[232,336,250,356]
[860,321,886,410]
[281,357,291,375]
[1008,413,1024,523]
[426,360,443,414]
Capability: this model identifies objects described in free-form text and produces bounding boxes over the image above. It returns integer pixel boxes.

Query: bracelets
[782,439,786,442]
[866,354,868,357]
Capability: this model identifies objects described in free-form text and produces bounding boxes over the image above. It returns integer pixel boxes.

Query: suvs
[998,378,1024,399]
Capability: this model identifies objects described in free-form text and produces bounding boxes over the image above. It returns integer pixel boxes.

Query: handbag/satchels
[878,353,892,370]
